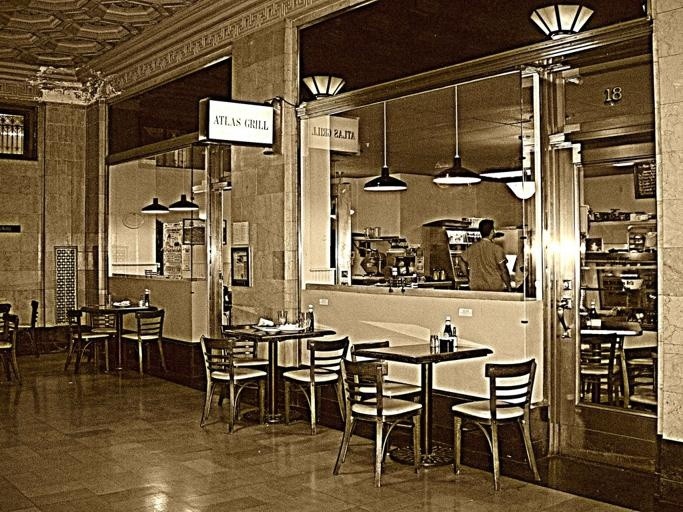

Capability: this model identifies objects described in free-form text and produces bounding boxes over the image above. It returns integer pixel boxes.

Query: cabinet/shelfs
[423,226,481,290]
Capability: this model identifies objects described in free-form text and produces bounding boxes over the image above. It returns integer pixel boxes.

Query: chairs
[0,300,38,386]
[578,318,658,408]
[63,303,166,377]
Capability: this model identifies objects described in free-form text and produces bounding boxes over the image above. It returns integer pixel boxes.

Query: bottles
[586,300,601,329]
[305,305,316,332]
[143,289,149,306]
[443,318,452,339]
[139,295,144,306]
[452,328,458,347]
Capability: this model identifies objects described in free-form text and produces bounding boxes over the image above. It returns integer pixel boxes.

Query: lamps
[530,0,595,39]
[141,155,169,214]
[433,85,482,185]
[302,76,346,99]
[363,102,408,192]
[169,149,199,211]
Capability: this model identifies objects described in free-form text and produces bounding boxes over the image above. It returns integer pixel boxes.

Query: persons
[459,219,513,290]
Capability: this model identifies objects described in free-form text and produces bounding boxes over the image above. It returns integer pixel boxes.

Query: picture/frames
[231,247,252,287]
[633,160,656,199]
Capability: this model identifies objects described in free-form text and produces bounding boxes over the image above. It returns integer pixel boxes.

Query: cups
[145,270,152,279]
[430,328,440,350]
[278,311,287,325]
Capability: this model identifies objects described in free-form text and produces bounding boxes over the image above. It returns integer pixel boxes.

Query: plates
[261,329,278,335]
[279,326,304,334]
